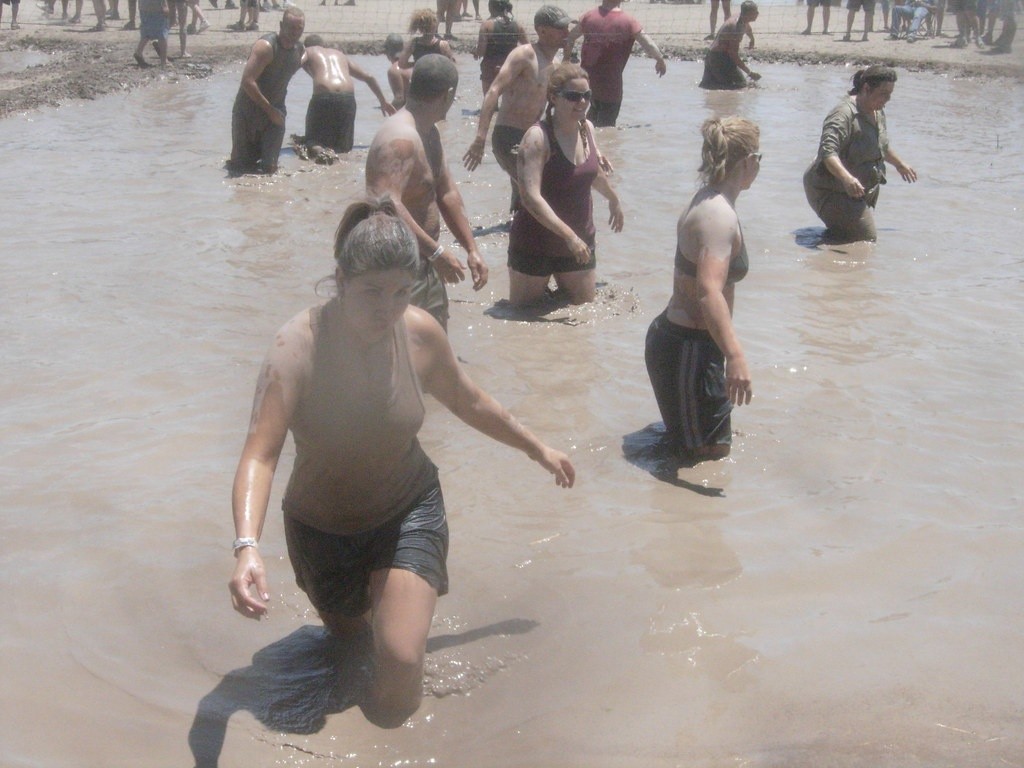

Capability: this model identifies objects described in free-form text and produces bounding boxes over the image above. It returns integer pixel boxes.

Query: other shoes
[474,14,482,20]
[443,32,457,40]
[883,35,899,41]
[843,35,850,41]
[948,38,968,47]
[273,4,282,11]
[121,21,135,29]
[226,20,259,30]
[703,34,714,41]
[282,2,297,7]
[68,15,82,24]
[907,35,914,43]
[11,20,20,30]
[975,37,985,48]
[343,1,356,5]
[86,22,109,32]
[462,12,473,18]
[821,30,833,36]
[209,0,218,9]
[105,8,120,21]
[197,20,210,32]
[36,3,54,14]
[224,1,238,10]
[862,33,868,41]
[803,29,811,35]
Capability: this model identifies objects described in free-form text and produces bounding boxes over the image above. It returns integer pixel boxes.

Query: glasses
[558,90,592,102]
[745,153,762,162]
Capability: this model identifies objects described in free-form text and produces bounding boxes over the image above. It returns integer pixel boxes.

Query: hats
[534,4,578,30]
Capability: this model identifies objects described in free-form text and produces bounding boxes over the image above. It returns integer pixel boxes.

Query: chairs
[899,0,939,40]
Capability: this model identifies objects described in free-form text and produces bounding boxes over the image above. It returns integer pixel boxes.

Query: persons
[0,0,1023,460]
[227,198,576,702]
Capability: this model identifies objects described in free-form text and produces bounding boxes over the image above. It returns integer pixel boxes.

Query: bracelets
[233,537,257,556]
[429,245,444,263]
[747,71,752,78]
